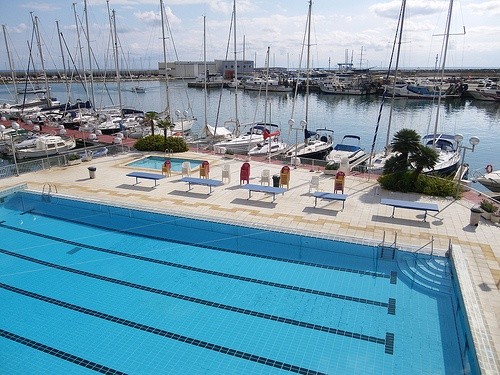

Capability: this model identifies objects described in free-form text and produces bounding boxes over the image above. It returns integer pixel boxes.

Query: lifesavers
[50,130,57,136]
[486,164,493,174]
[274,138,278,143]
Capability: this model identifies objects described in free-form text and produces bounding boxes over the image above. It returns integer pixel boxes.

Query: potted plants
[325,159,340,176]
[481,199,500,227]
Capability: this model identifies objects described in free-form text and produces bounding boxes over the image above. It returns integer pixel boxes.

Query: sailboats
[404,0,480,185]
[215,46,279,155]
[176,16,238,150]
[350,0,416,175]
[280,0,337,167]
[123,1,179,139]
[0,0,150,136]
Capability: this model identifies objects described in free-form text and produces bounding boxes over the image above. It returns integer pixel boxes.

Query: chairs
[181,161,191,179]
[334,171,346,195]
[223,164,231,185]
[200,160,210,179]
[238,162,251,186]
[308,175,319,193]
[260,165,290,189]
[161,160,172,178]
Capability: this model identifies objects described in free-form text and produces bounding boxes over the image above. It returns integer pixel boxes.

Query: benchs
[182,177,223,196]
[127,171,167,189]
[380,198,439,221]
[243,183,286,205]
[313,191,348,212]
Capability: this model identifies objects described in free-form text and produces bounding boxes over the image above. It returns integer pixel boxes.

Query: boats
[325,135,370,171]
[187,72,229,88]
[294,61,500,104]
[247,136,288,158]
[13,135,80,159]
[237,71,292,91]
[132,85,146,93]
[0,126,38,154]
[474,170,500,191]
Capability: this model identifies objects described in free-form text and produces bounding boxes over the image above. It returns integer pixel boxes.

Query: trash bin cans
[470,207,483,226]
[89,166,96,179]
